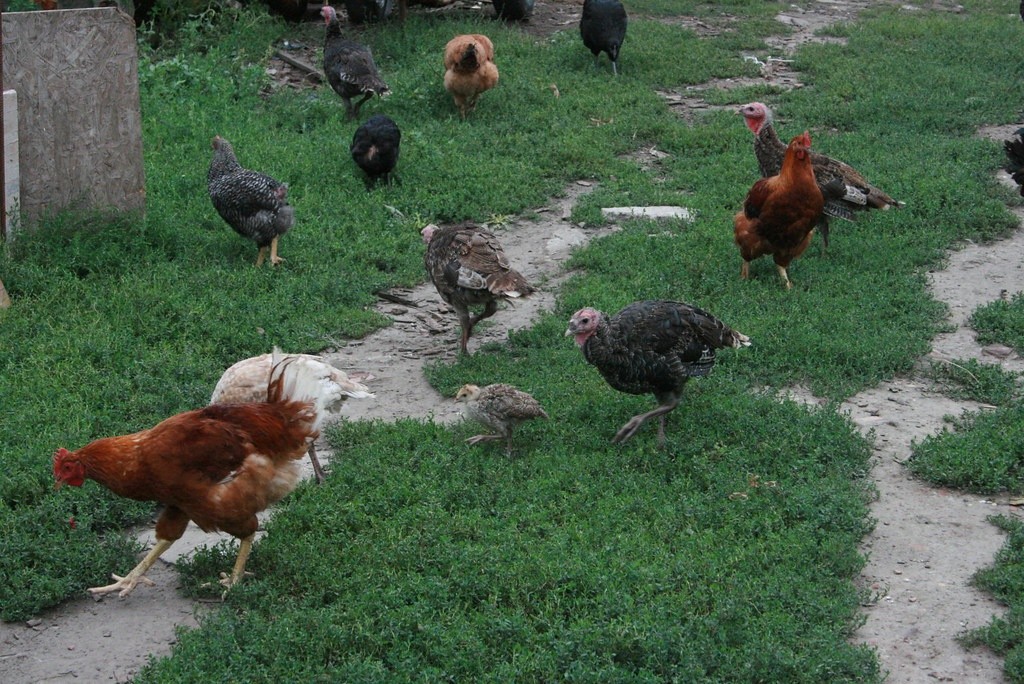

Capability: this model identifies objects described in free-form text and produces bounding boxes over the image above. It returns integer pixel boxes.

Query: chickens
[320,5,393,122]
[442,35,498,120]
[419,223,537,359]
[207,353,377,485]
[579,0,628,75]
[350,117,401,187]
[53,344,335,603]
[564,301,753,445]
[733,130,824,293]
[453,383,549,453]
[733,101,907,260]
[207,134,294,265]
